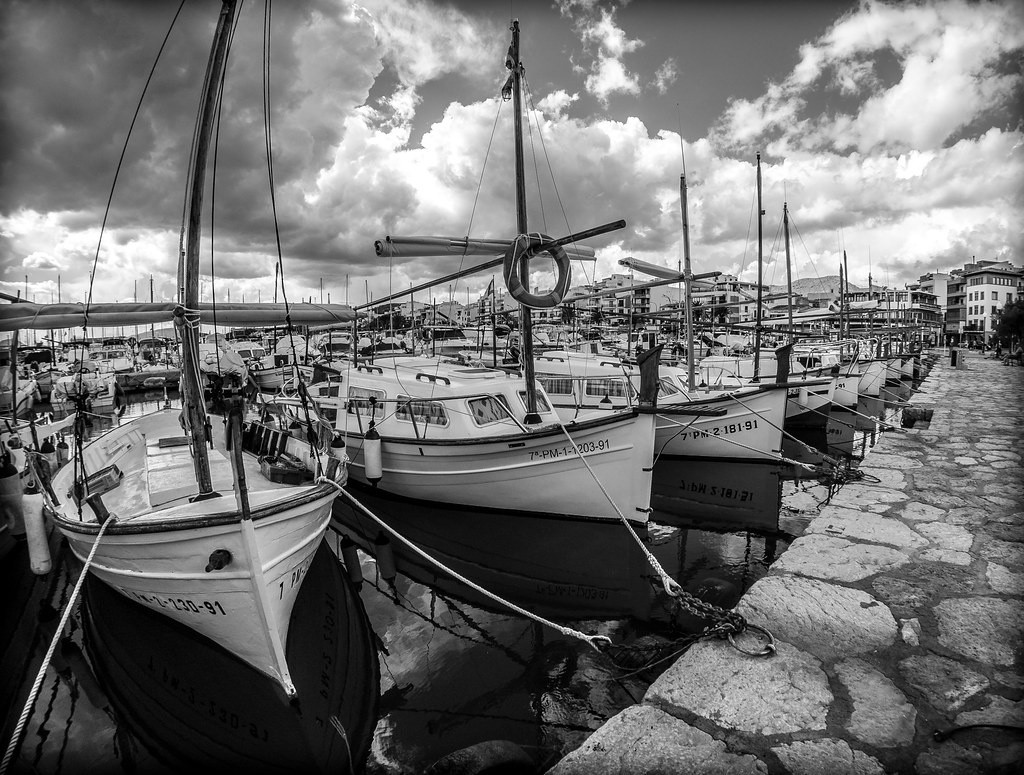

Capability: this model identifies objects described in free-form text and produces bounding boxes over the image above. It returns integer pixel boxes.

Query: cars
[968,340,991,351]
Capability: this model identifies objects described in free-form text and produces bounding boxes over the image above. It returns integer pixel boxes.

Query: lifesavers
[503,231,575,311]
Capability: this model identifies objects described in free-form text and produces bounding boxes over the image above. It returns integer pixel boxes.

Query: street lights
[982,313,986,353]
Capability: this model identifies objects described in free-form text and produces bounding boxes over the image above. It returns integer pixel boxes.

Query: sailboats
[1,275,519,396]
[333,483,685,749]
[72,539,383,775]
[536,150,928,461]
[22,0,348,699]
[651,456,784,541]
[258,19,664,523]
